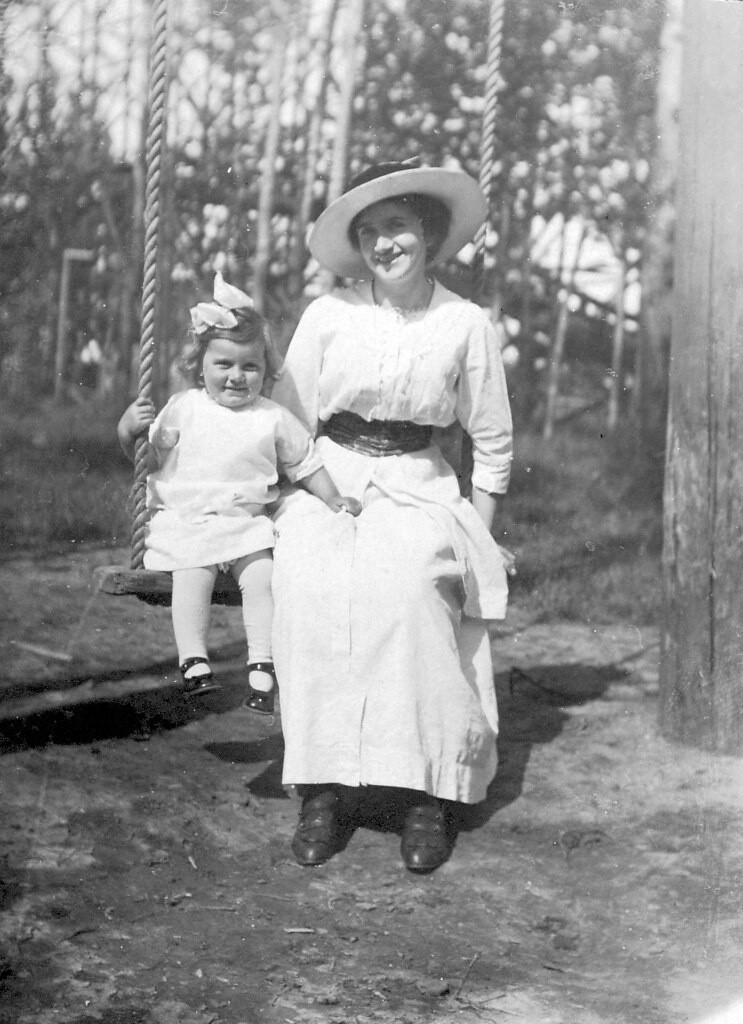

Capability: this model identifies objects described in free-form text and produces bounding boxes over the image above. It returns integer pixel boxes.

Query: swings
[85,0,518,609]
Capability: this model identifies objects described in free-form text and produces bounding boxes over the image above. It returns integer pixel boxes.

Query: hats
[306,163,487,281]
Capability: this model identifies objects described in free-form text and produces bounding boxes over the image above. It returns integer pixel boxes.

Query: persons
[116,269,364,715]
[267,160,519,873]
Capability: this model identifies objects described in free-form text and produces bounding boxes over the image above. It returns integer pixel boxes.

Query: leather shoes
[400,792,456,873]
[292,789,340,867]
[181,657,219,696]
[243,663,275,714]
